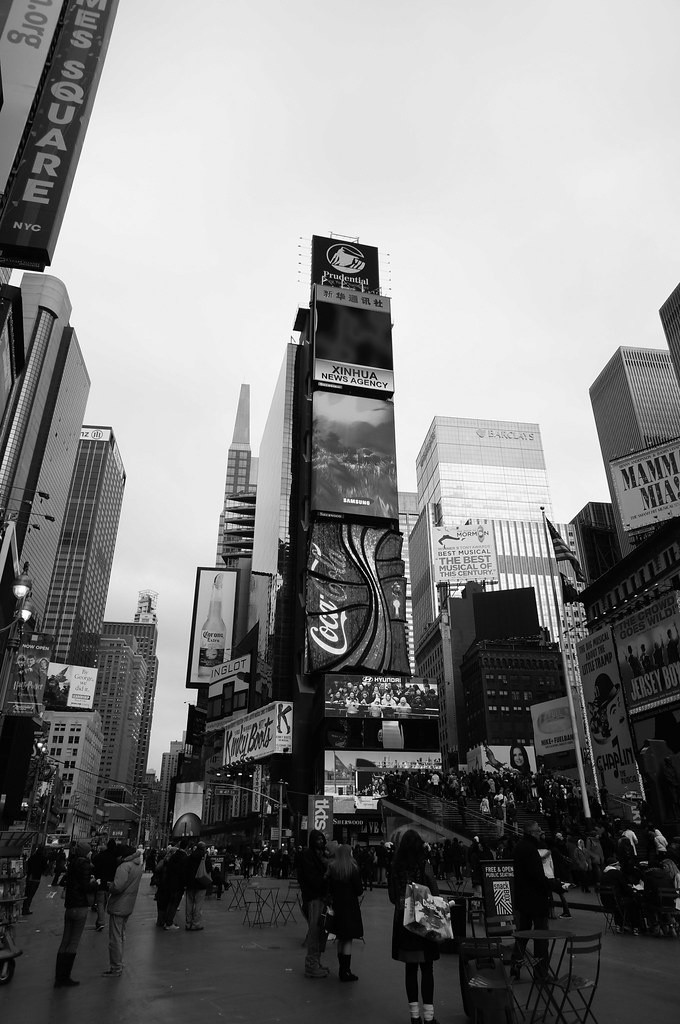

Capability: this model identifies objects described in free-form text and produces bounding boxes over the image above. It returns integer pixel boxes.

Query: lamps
[12,561,32,600]
[14,592,34,623]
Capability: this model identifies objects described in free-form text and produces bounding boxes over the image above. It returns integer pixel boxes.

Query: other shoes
[651,926,663,936]
[97,924,105,931]
[22,910,33,915]
[225,883,232,890]
[616,924,623,934]
[667,928,677,937]
[92,904,98,911]
[190,923,204,930]
[632,927,639,935]
[186,924,191,929]
[559,913,572,919]
[54,977,80,988]
[102,968,122,976]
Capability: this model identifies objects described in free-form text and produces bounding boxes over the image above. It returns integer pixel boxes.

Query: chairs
[460,887,680,1024]
[227,877,302,928]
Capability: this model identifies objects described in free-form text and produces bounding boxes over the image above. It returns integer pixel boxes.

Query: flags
[559,571,580,603]
[546,518,585,583]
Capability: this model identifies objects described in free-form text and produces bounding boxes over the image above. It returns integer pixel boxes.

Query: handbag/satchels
[194,852,213,886]
[150,871,161,885]
[402,860,455,942]
[484,806,488,812]
[323,914,336,933]
[59,875,66,886]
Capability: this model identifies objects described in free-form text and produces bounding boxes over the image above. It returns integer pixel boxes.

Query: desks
[254,886,280,930]
[511,931,572,1024]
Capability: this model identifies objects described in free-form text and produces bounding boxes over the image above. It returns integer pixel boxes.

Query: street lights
[0,562,34,714]
[25,742,46,831]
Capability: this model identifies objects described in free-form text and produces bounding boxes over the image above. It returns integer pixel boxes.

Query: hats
[325,840,338,856]
[75,842,91,857]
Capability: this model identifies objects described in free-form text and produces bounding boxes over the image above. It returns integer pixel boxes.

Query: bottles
[198,584,226,676]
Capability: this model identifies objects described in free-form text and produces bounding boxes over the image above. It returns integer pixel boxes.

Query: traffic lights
[206,788,212,799]
[74,796,80,806]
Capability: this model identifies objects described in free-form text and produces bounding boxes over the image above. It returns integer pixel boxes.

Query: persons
[142,839,292,930]
[102,845,143,977]
[510,746,530,775]
[394,757,441,768]
[385,767,680,979]
[508,821,571,983]
[327,678,437,719]
[20,840,145,929]
[389,829,443,1023]
[624,623,680,677]
[52,840,106,988]
[355,772,387,796]
[294,832,388,982]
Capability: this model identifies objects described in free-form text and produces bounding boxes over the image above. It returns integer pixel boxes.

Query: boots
[338,952,358,982]
[305,955,330,976]
[423,1004,434,1024]
[408,1002,421,1022]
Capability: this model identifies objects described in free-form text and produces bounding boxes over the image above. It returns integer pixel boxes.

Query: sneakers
[164,923,180,929]
